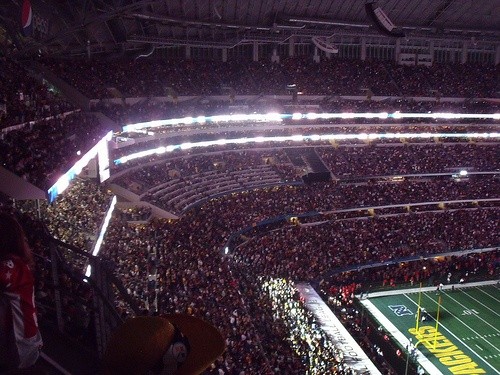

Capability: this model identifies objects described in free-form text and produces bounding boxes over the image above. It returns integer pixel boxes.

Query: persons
[0,53,500,375]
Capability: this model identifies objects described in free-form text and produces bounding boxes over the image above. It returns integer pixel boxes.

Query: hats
[99,311,225,375]
